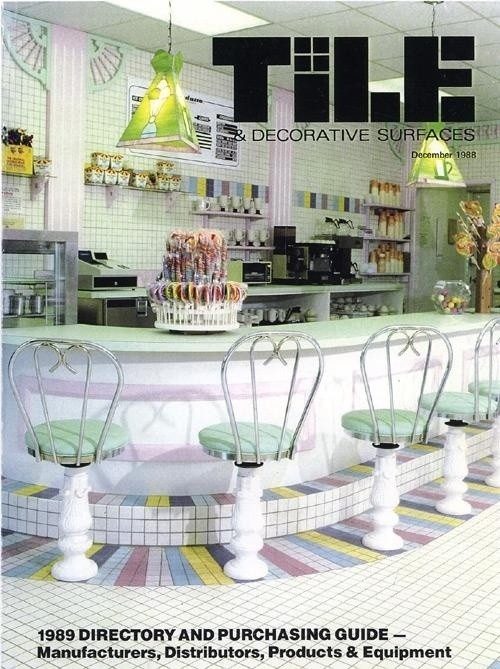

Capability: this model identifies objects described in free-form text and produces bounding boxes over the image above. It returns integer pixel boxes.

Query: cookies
[226,259,273,285]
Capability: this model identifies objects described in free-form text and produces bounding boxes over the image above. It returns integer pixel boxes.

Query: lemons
[336,218,354,237]
[287,306,301,322]
[321,216,336,235]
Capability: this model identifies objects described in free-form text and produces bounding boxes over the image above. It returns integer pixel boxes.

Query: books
[1,144,34,179]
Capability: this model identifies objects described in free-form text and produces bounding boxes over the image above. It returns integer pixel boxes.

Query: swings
[272,226,309,285]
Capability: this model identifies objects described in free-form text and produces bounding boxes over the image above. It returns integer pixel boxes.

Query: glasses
[115,2,201,154]
[406,2,467,189]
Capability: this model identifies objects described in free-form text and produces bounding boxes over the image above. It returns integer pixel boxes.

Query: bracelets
[330,296,397,320]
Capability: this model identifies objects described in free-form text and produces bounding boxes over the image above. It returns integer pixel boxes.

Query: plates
[359,189,417,312]
[1,228,79,325]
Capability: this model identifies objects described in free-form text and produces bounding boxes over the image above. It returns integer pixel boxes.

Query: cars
[400,214,405,238]
[219,196,230,209]
[369,251,377,262]
[385,183,389,205]
[196,198,210,211]
[386,251,390,273]
[394,252,400,272]
[389,184,393,205]
[268,308,278,321]
[279,308,285,322]
[378,212,386,236]
[398,251,403,273]
[388,214,395,236]
[259,229,269,241]
[247,230,258,243]
[379,183,385,205]
[384,207,390,218]
[209,197,217,211]
[264,310,268,320]
[255,197,264,210]
[391,250,395,273]
[371,180,379,204]
[378,255,386,272]
[256,309,264,321]
[377,242,403,254]
[231,196,242,208]
[243,198,254,209]
[9,292,26,315]
[396,184,402,206]
[235,229,245,241]
[395,211,400,237]
[28,291,45,314]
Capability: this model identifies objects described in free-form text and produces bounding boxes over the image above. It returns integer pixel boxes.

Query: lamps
[340,325,454,551]
[8,337,127,582]
[418,316,500,516]
[198,331,324,580]
[465,380,500,491]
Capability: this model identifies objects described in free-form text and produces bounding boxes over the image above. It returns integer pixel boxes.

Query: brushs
[316,235,363,284]
[299,239,340,285]
